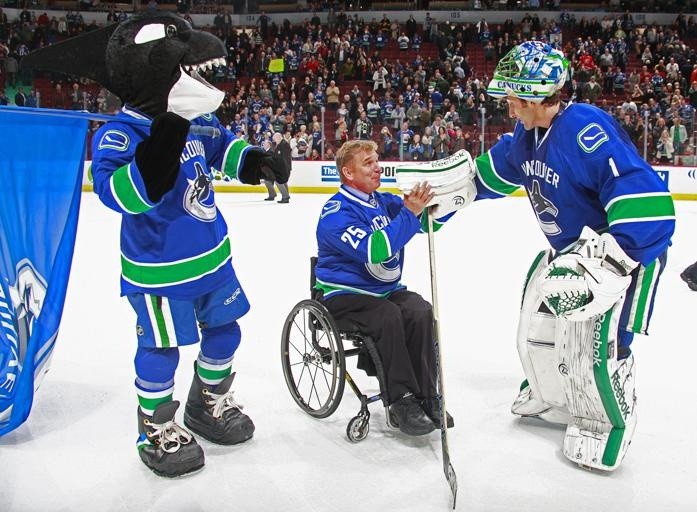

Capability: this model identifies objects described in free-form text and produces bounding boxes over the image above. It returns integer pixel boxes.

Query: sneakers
[388,393,437,437]
[136,400,206,482]
[182,359,256,447]
[264,196,291,204]
[416,391,456,431]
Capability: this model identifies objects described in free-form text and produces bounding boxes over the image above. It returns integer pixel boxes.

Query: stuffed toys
[20,12,289,479]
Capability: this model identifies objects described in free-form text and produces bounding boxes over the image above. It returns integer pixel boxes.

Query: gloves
[536,224,642,324]
[392,147,480,222]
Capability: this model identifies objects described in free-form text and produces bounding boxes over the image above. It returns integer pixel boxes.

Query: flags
[0,109,88,438]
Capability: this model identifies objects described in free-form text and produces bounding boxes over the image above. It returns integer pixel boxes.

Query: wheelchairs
[276,253,453,446]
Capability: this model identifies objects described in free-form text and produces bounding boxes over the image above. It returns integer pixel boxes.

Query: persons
[394,37,675,472]
[315,138,454,436]
[273,131,291,203]
[1,0,697,167]
[261,141,277,200]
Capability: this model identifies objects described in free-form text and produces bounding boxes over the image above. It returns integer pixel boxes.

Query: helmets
[486,39,571,105]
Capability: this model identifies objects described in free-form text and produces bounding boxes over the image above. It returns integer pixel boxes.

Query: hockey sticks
[426,205,458,510]
